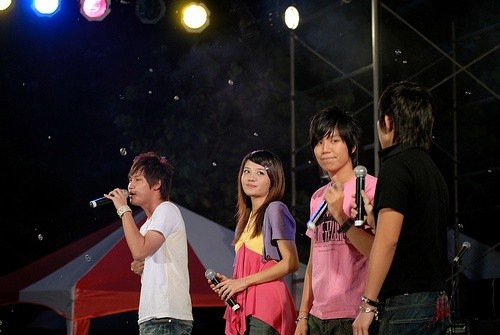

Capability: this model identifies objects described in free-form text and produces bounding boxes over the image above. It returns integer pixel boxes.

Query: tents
[2,195,307,334]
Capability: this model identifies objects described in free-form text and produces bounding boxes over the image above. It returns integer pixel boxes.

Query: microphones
[354,166,367,227]
[90,190,128,208]
[454,242,471,262]
[307,183,344,231]
[205,269,240,312]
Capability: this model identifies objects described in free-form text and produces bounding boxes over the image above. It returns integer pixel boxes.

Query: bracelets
[359,305,379,321]
[297,310,309,316]
[295,316,308,326]
[361,295,379,308]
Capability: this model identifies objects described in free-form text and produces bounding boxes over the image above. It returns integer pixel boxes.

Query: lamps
[266,3,302,33]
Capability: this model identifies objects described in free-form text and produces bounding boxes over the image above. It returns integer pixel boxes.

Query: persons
[103,151,201,335]
[208,149,298,335]
[293,104,378,334]
[351,78,453,335]
